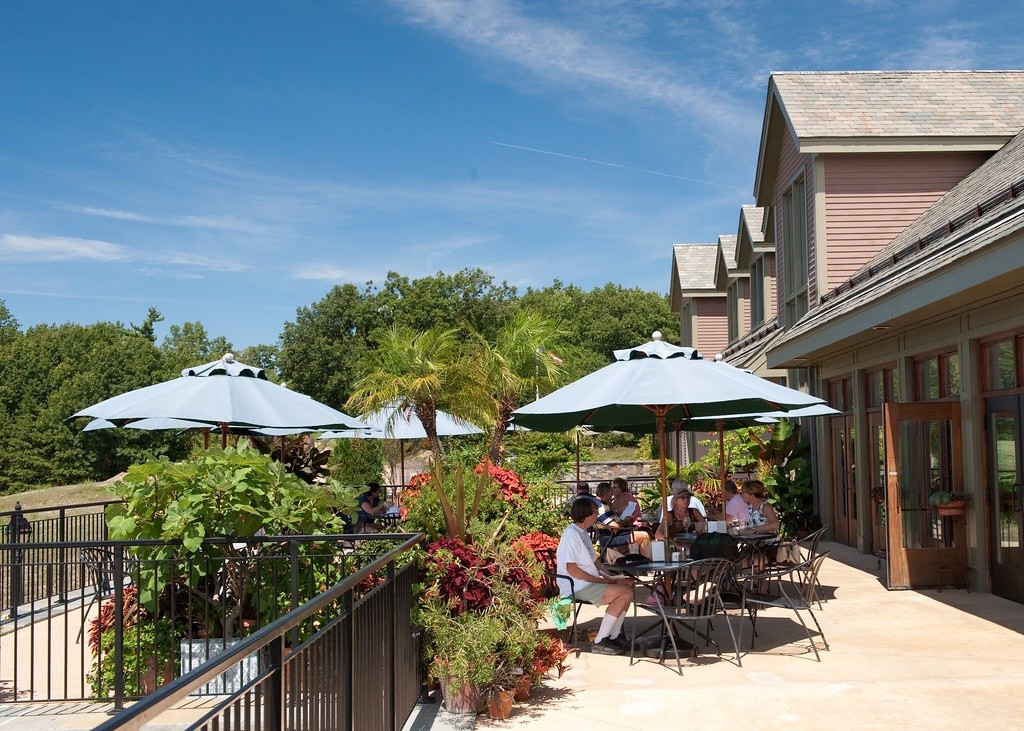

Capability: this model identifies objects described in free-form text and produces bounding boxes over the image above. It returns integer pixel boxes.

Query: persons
[556,497,634,655]
[646,480,781,604]
[355,483,393,534]
[568,478,652,561]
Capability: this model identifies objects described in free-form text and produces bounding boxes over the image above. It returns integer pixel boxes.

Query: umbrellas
[506,331,843,595]
[60,354,380,449]
[318,398,486,492]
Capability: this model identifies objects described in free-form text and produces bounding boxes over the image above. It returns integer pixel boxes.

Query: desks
[673,533,779,600]
[637,517,657,527]
[365,512,400,519]
[604,560,730,656]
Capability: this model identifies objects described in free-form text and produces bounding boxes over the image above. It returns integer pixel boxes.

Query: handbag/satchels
[689,531,740,565]
[776,520,801,567]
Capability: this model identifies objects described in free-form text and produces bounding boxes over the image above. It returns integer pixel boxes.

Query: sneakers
[591,635,622,655]
[610,633,640,651]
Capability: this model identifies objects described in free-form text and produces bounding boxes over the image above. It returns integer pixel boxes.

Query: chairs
[74,548,138,643]
[534,520,833,673]
[353,513,402,533]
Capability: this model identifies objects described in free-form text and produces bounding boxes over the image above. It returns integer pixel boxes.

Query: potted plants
[869,486,886,527]
[930,492,969,516]
[86,441,373,698]
[410,581,532,719]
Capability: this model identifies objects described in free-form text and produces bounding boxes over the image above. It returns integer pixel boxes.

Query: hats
[674,489,694,499]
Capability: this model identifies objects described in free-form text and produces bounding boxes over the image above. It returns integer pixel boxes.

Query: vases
[797,531,812,541]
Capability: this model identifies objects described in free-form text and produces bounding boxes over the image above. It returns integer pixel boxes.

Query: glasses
[676,495,691,501]
[613,485,621,489]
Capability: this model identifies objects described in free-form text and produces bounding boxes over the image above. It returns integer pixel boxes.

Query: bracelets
[752,527,757,534]
